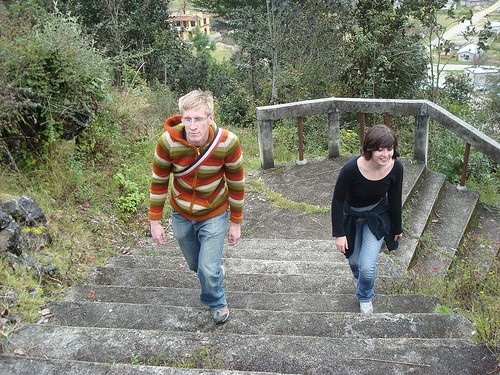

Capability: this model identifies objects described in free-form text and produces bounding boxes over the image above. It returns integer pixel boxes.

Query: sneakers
[359,299,373,314]
[210,304,230,324]
[353,276,358,288]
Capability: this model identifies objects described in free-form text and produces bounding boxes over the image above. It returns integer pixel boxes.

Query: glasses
[181,113,211,123]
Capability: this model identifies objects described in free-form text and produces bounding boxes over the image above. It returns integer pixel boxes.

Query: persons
[149,90,244,324]
[331,124,402,315]
